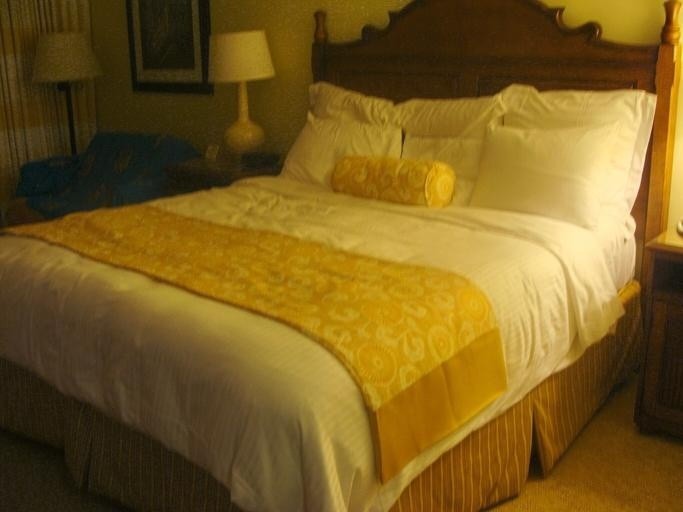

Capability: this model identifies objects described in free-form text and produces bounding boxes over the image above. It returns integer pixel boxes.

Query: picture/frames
[127,0,213,95]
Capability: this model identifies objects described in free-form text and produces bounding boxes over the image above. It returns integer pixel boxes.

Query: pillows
[280,109,401,190]
[306,80,392,125]
[503,83,658,244]
[330,154,457,213]
[469,123,613,240]
[381,94,503,208]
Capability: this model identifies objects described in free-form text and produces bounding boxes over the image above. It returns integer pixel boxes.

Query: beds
[3,0,682,511]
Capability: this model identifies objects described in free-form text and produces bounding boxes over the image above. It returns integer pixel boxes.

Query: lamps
[31,29,104,156]
[208,26,275,152]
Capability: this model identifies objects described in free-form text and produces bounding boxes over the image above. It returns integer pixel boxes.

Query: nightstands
[633,231,683,437]
[169,162,277,189]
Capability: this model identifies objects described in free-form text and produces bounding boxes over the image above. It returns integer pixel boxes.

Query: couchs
[14,130,197,218]
